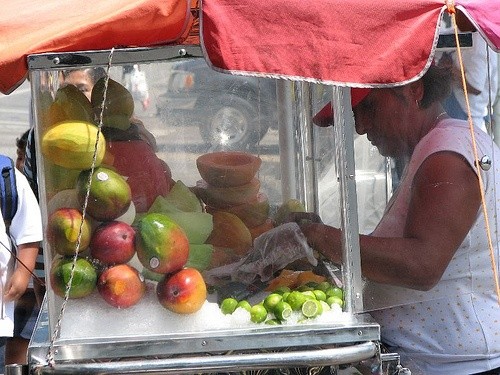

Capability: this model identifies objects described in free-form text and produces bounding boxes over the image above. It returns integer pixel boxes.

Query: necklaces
[424,110,448,136]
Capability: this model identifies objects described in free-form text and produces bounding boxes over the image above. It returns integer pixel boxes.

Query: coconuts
[45,84,95,126]
[91,75,135,132]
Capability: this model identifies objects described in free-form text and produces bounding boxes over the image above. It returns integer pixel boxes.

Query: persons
[278,57,499,375]
[442,9,497,136]
[8,56,175,375]
[0,152,42,374]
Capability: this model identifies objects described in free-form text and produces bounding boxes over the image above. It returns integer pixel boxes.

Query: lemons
[221,282,343,325]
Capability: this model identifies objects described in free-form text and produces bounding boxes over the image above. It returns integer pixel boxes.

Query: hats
[313,86,373,127]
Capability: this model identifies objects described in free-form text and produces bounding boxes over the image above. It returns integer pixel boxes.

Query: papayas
[76,167,133,222]
[156,268,207,315]
[90,220,138,264]
[134,212,189,273]
[42,118,106,170]
[47,207,92,256]
[194,151,304,256]
[97,263,146,309]
[266,269,326,292]
[49,256,97,300]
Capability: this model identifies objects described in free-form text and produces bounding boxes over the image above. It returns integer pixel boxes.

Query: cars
[157,56,279,149]
